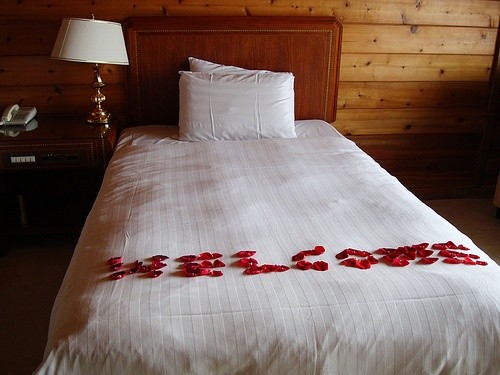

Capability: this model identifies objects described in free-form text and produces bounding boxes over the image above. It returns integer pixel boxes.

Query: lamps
[51,13,129,124]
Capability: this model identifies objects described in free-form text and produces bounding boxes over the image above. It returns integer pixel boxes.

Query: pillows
[188,56,270,72]
[178,70,294,142]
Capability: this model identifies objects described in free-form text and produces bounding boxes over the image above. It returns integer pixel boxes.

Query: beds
[32,15,500,375]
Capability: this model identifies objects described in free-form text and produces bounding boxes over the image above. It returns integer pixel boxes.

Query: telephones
[0,103,38,126]
[0,119,38,137]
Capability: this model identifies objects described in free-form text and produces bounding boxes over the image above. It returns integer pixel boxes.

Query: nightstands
[0,119,119,257]
